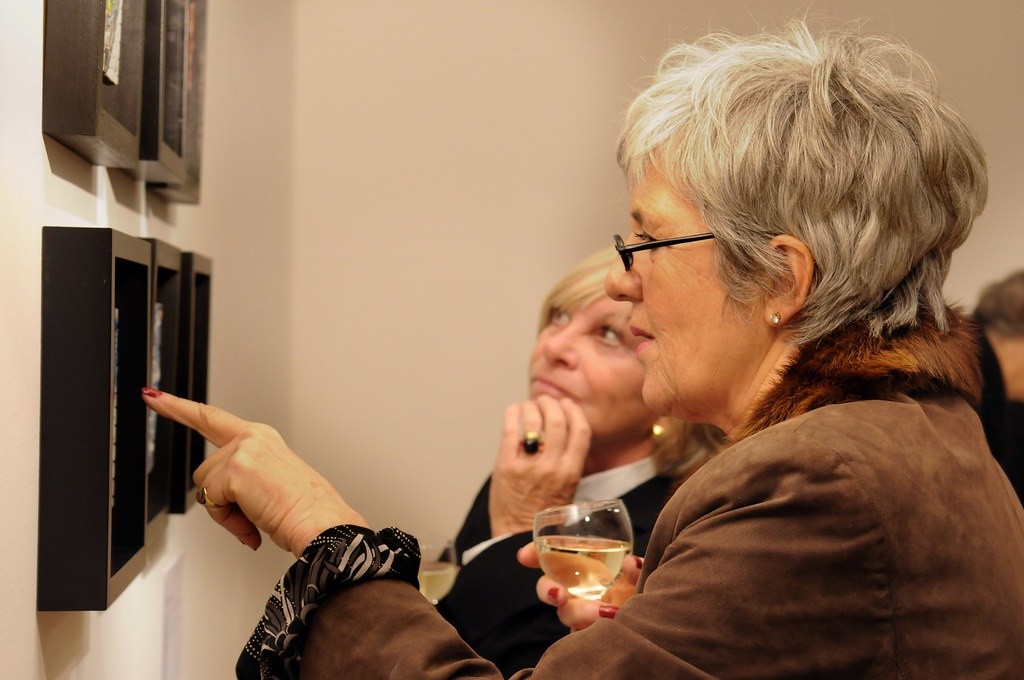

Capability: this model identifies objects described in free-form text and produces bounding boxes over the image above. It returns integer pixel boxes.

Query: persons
[141,15,1024,680]
[971,269,1024,402]
[431,240,676,680]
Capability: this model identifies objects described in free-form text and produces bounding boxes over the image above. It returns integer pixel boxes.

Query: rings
[196,487,221,508]
[519,432,544,455]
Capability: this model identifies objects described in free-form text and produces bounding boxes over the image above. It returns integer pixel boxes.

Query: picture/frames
[43,0,207,203]
[37,227,212,612]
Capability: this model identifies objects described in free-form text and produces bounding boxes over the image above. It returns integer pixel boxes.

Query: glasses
[613,233,716,271]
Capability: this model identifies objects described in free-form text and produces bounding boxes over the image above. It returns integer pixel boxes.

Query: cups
[535,497,635,602]
[414,539,458,605]
